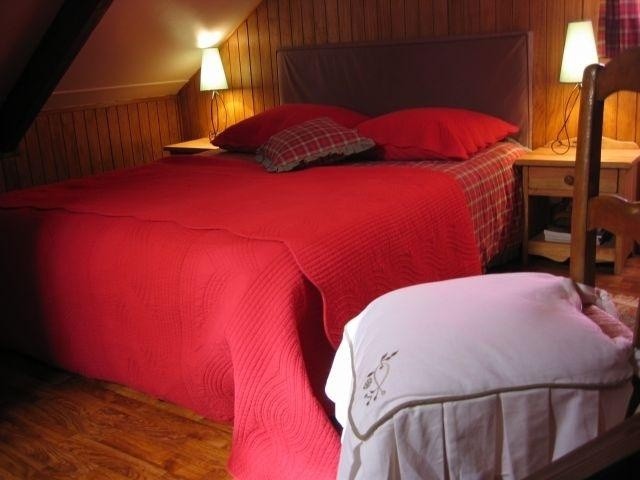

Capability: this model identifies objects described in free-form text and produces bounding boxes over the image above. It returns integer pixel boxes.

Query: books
[545,218,606,245]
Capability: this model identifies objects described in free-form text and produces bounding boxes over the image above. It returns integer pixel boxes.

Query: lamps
[199,46,229,142]
[550,20,600,156]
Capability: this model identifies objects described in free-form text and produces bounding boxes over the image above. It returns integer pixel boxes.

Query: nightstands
[513,136,639,277]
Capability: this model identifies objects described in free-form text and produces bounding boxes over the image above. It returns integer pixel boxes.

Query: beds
[3,37,529,430]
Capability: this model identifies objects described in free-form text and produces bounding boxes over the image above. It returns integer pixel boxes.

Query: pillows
[255,116,376,173]
[210,101,371,153]
[352,101,519,161]
